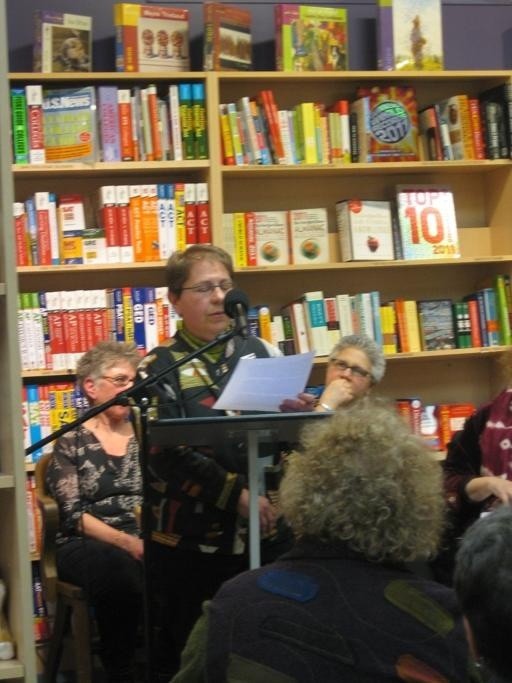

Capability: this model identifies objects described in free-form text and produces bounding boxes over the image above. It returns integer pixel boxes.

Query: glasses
[330,356,374,383]
[100,373,135,386]
[180,280,235,296]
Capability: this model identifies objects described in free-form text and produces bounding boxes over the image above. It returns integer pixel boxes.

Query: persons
[130,244,293,681]
[172,412,484,683]
[316,403,334,412]
[304,334,387,413]
[446,504,511,681]
[444,389,511,521]
[45,340,194,683]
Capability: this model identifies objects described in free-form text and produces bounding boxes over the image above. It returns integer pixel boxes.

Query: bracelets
[114,531,126,544]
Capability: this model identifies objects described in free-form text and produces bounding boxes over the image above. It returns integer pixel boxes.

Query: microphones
[224,290,249,339]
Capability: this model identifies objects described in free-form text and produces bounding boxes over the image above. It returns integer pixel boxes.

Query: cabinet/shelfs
[7,70,214,683]
[1,0,32,683]
[212,69,512,466]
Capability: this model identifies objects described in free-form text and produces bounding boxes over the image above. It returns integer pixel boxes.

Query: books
[32,8,93,73]
[12,182,212,267]
[220,82,512,164]
[114,1,193,72]
[24,382,92,460]
[26,472,49,644]
[392,183,461,261]
[11,80,212,164]
[243,270,511,355]
[274,3,350,71]
[375,0,445,73]
[395,397,480,453]
[18,287,183,370]
[200,2,254,72]
[224,182,437,265]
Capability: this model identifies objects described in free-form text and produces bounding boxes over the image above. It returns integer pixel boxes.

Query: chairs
[30,452,101,683]
